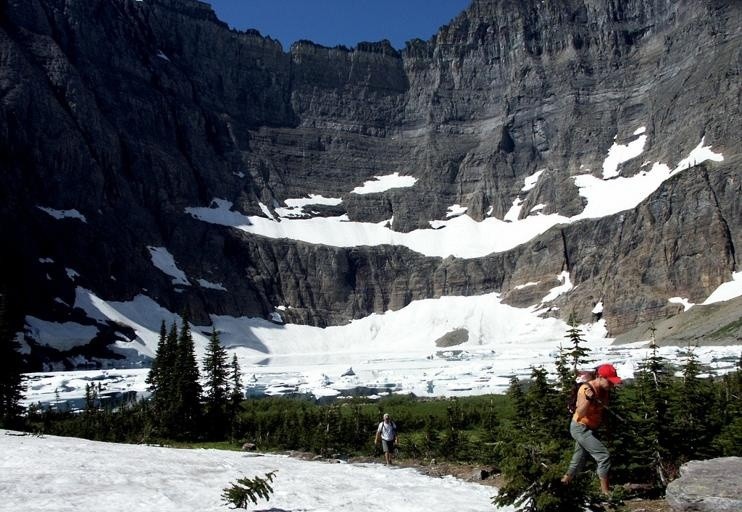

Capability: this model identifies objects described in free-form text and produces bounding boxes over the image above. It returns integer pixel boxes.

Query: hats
[384,414,390,419]
[597,364,621,384]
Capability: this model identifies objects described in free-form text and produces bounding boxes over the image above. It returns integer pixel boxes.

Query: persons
[560,364,624,496]
[374,413,399,468]
